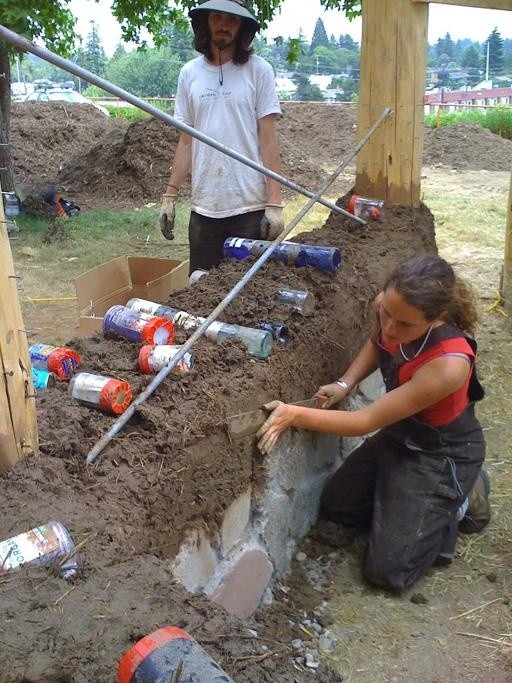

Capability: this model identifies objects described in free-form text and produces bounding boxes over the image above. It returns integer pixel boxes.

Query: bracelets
[333,381,350,393]
[166,183,180,192]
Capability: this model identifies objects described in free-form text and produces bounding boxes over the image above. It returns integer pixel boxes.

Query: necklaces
[400,324,433,362]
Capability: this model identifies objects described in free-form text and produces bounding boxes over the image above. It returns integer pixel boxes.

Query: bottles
[1,519,81,583]
[275,288,315,316]
[137,344,193,373]
[195,317,270,359]
[188,270,210,288]
[347,195,385,220]
[101,304,174,345]
[27,341,82,390]
[64,373,132,413]
[117,625,236,682]
[222,234,342,272]
[125,297,196,333]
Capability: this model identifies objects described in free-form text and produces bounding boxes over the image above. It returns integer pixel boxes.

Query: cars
[10,78,57,96]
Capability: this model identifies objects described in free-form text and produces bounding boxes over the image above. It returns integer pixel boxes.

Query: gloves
[259,203,285,241]
[159,193,178,241]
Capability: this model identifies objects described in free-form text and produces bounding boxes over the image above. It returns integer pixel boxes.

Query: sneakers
[458,468,492,535]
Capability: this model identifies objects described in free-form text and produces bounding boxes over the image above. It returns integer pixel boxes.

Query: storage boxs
[70,254,191,337]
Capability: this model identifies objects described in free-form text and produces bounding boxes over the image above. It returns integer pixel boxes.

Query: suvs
[22,81,111,118]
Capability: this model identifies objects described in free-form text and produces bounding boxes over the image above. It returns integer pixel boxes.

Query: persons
[158,0,286,278]
[257,255,492,596]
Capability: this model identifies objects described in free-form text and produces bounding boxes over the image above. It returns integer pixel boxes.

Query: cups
[260,318,289,345]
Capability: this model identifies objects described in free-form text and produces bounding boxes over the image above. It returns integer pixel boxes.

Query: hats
[188,0,258,28]
